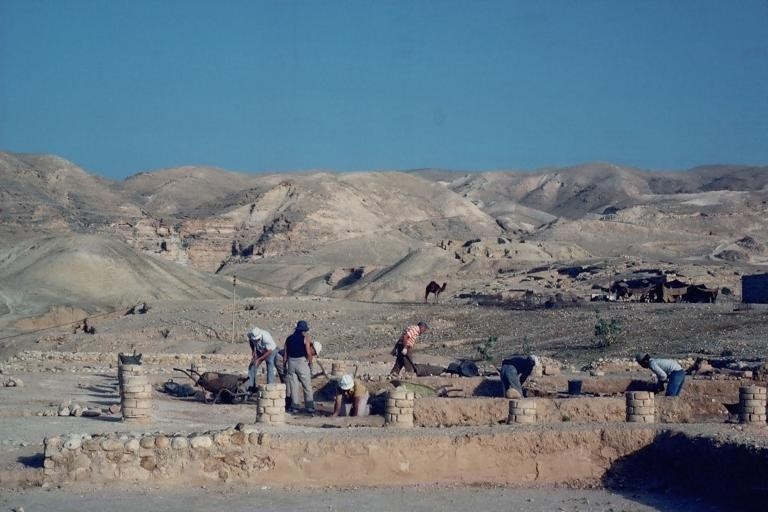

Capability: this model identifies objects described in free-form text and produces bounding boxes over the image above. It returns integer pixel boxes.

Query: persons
[246,327,278,385]
[389,322,430,374]
[331,374,370,416]
[634,351,685,396]
[283,320,316,413]
[500,354,540,396]
[274,340,323,397]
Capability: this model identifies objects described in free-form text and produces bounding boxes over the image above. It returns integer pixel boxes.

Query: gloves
[401,347,408,356]
[391,348,396,356]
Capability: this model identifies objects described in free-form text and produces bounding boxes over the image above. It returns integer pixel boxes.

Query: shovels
[246,345,259,397]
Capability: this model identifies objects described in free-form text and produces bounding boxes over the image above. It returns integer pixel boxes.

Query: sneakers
[293,407,300,414]
[306,408,315,414]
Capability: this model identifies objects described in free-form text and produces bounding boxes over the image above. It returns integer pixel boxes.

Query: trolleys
[406,354,446,376]
[173,368,250,403]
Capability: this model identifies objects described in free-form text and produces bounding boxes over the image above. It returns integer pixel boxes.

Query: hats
[530,355,538,364]
[636,351,648,363]
[250,327,263,341]
[337,374,354,390]
[295,320,308,331]
[312,341,322,357]
